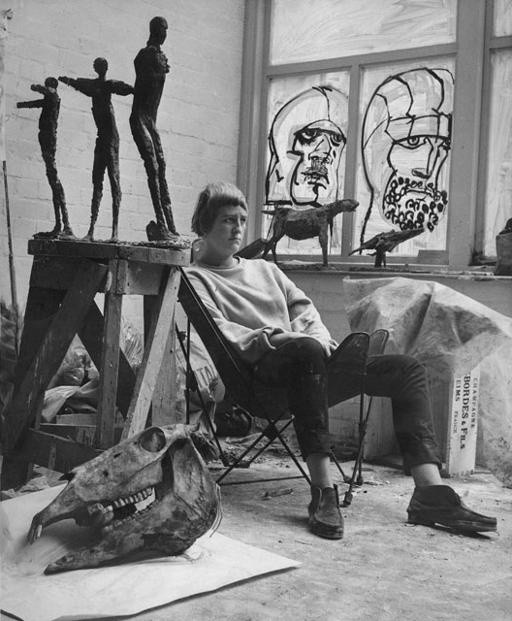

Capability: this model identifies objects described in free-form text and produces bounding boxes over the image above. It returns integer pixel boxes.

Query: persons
[173,181,498,541]
[267,82,344,227]
[130,18,180,238]
[357,64,454,254]
[59,57,137,246]
[17,76,73,238]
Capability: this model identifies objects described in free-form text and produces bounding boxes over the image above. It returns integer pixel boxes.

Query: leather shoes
[407,484,498,532]
[308,484,344,538]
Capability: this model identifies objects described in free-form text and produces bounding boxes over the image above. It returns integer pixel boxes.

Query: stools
[1,238,192,491]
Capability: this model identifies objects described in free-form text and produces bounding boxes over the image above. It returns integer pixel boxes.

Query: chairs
[143,219,390,507]
